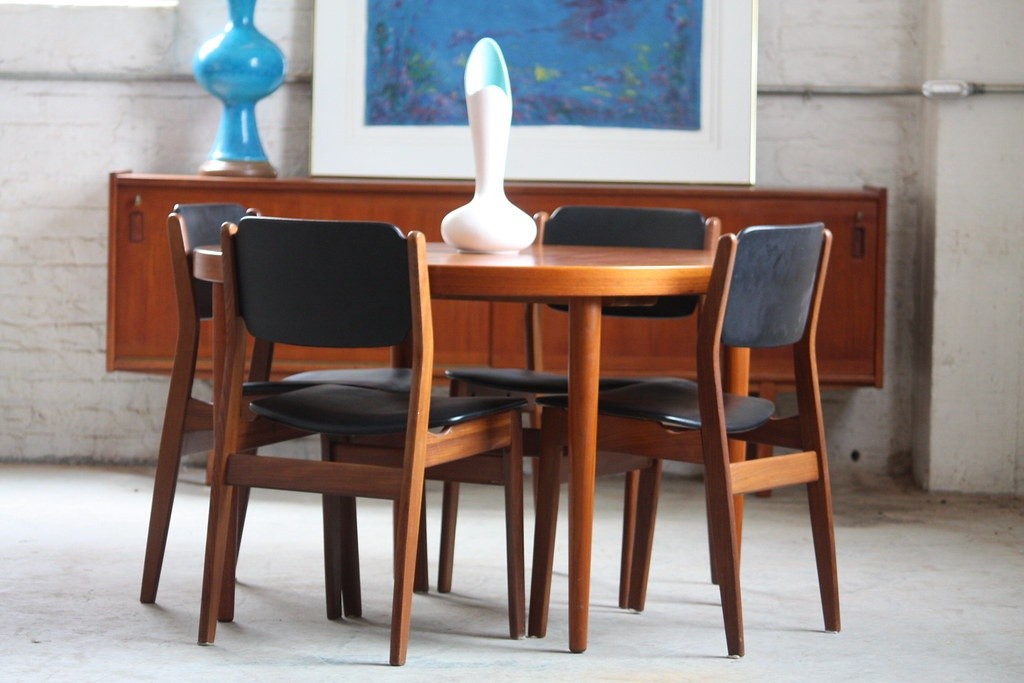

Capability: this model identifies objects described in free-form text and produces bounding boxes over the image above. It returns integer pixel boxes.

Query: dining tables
[188,245,750,654]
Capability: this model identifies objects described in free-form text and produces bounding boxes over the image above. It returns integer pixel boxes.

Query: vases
[438,36,538,254]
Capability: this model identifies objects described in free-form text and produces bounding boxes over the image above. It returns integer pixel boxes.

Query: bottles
[192,0,287,178]
[441,37,538,255]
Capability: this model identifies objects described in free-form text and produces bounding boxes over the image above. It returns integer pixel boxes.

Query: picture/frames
[308,0,759,187]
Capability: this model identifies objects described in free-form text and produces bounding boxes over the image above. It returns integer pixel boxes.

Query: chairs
[139,203,430,620]
[435,203,725,613]
[197,216,532,666]
[530,223,842,661]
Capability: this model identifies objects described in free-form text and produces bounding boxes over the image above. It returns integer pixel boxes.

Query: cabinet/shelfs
[109,171,897,377]
